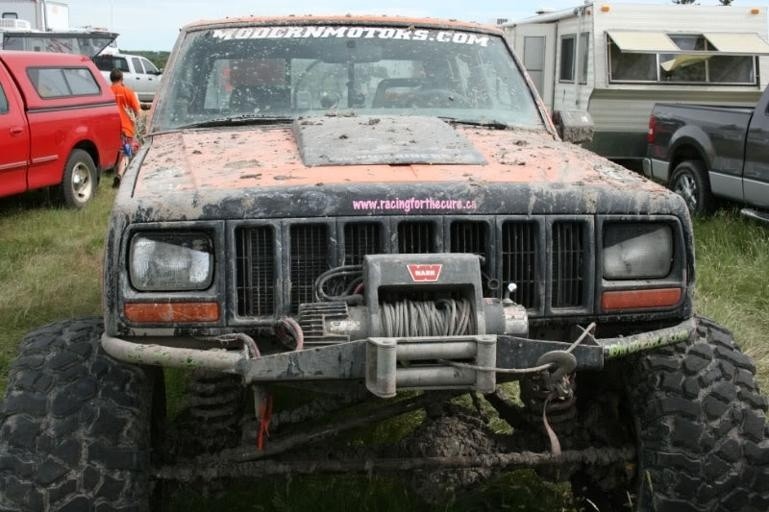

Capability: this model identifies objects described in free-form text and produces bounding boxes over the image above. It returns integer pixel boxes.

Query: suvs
[102,15,769,512]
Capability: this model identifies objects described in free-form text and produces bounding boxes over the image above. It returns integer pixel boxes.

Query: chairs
[370,77,432,109]
[230,83,291,114]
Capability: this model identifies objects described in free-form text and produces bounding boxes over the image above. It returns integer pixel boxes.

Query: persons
[110,69,141,188]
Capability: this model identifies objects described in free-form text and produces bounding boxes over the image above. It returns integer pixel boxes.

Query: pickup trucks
[640,83,769,223]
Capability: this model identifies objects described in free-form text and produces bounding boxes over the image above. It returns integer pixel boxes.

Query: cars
[0,49,163,209]
